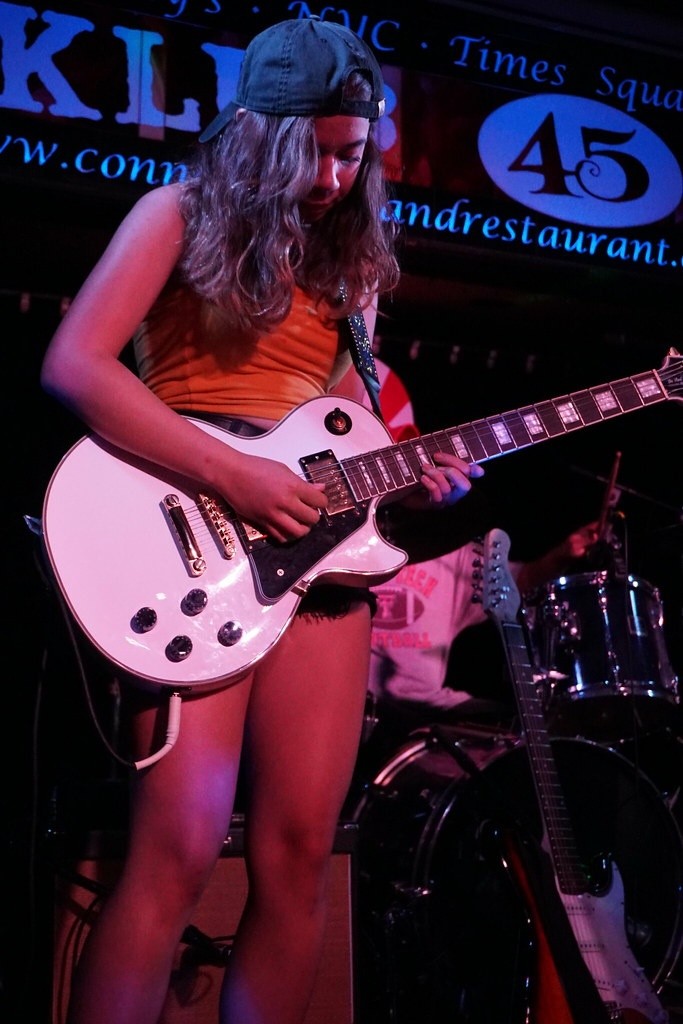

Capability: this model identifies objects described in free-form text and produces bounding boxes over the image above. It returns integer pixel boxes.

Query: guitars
[31,343,682,708]
[460,521,669,1021]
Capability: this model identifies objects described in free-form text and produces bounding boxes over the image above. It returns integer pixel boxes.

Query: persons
[44,14,484,1024]
[368,522,611,716]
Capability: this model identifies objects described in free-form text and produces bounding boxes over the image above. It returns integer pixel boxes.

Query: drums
[521,569,680,746]
[347,725,683,1023]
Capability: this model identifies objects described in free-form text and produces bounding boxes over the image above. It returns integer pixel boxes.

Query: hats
[200,14,385,144]
